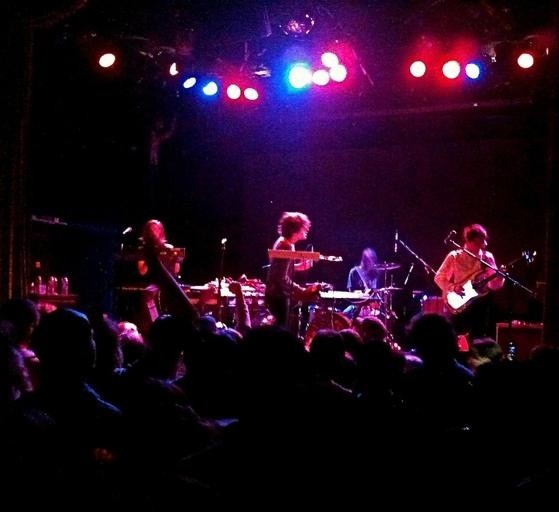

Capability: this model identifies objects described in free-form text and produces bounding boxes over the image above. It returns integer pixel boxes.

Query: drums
[420,296,447,317]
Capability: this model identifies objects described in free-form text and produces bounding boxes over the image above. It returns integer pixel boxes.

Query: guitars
[228,284,251,337]
[292,281,332,308]
[442,251,538,314]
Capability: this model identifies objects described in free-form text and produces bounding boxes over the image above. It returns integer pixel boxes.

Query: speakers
[496,323,544,360]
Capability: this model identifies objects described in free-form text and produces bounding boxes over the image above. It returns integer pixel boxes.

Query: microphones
[221,237,227,244]
[122,226,132,235]
[394,228,399,253]
[443,229,456,245]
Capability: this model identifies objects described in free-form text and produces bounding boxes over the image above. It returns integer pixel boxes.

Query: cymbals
[372,287,401,291]
[373,263,400,269]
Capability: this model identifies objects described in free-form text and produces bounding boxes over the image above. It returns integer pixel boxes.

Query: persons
[434,224,514,329]
[1,282,558,512]
[264,212,317,326]
[342,248,385,325]
[138,219,180,330]
